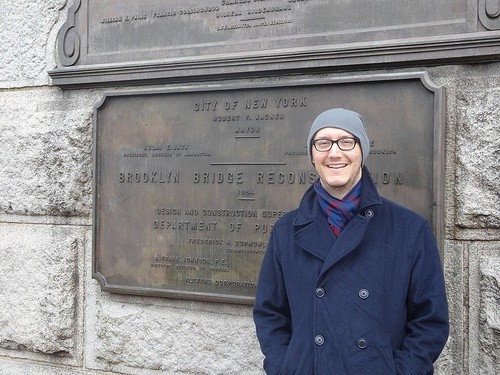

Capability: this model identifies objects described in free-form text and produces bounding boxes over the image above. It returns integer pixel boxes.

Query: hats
[307,107,370,168]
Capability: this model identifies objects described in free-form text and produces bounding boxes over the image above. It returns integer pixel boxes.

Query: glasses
[310,137,359,152]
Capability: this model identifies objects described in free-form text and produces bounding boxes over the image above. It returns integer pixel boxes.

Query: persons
[254,107,449,375]
